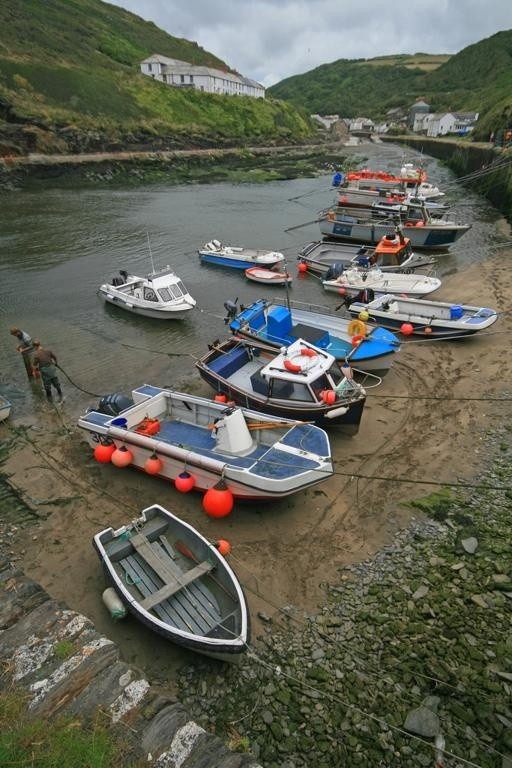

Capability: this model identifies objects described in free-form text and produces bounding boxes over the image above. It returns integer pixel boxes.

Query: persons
[30,340,64,398]
[9,326,34,383]
[224,300,237,325]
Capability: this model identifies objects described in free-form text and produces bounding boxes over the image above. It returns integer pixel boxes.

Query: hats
[10,327,18,335]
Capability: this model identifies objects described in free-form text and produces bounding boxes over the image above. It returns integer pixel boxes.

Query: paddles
[208,421,295,430]
[173,540,237,604]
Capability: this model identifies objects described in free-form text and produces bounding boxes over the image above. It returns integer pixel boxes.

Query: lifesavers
[283,349,318,371]
[405,223,412,227]
[382,235,400,245]
